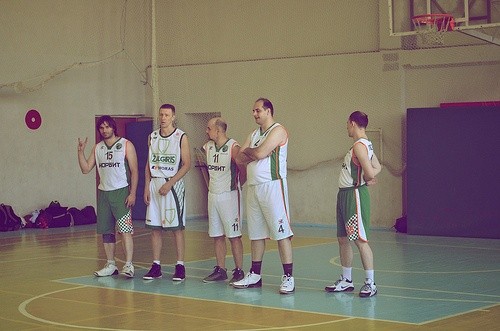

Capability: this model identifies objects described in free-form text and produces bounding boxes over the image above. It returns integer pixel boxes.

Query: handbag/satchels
[26,202,97,228]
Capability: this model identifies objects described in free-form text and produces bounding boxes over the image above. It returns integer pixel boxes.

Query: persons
[77,115,138,277]
[325,111,381,298]
[200,118,247,285]
[143,104,192,281]
[232,98,295,294]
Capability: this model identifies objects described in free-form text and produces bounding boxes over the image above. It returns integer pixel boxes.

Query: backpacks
[0,203,24,231]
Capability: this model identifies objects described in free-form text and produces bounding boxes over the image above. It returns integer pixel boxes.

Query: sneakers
[172,264,185,281]
[93,263,119,277]
[143,263,162,280]
[229,268,244,285]
[233,271,262,289]
[279,275,295,294]
[359,278,377,297]
[203,266,229,283]
[120,264,136,278]
[324,275,354,292]
